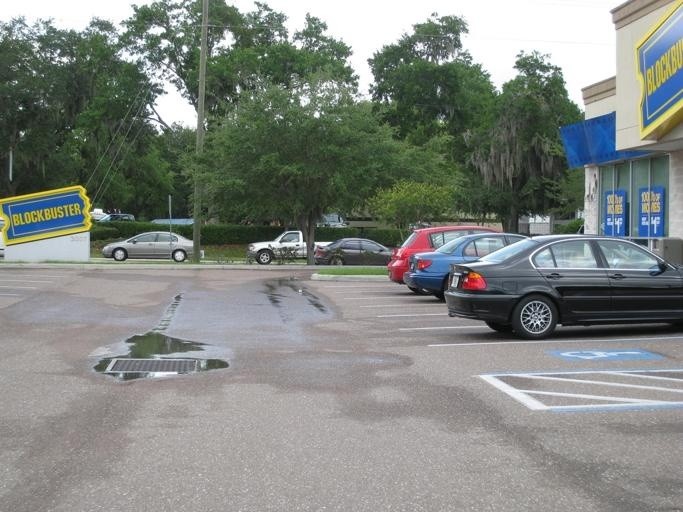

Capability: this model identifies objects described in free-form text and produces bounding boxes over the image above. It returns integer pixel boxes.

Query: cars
[315,213,350,229]
[386,223,683,340]
[88,208,136,223]
[311,238,394,266]
[101,233,197,264]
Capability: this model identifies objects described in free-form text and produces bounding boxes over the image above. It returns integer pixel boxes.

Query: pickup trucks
[249,231,337,265]
